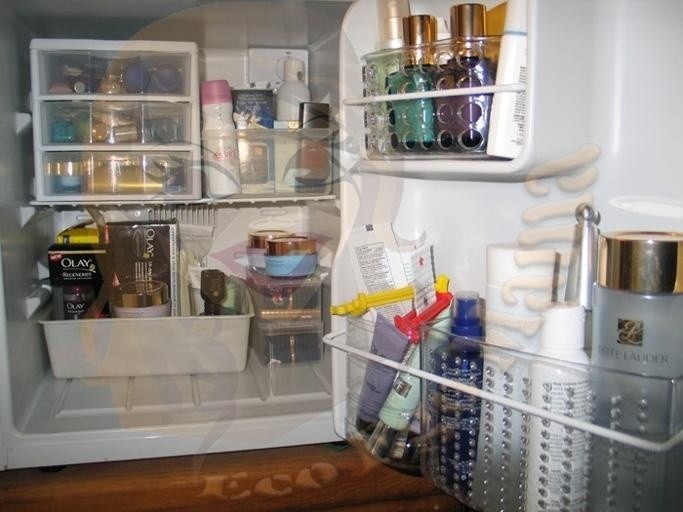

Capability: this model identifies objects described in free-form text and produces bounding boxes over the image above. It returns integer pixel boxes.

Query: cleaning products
[483,0,528,158]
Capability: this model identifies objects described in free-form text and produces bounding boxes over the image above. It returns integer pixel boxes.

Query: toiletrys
[264,237,317,278]
[28,0,204,202]
[201,55,334,202]
[417,203,682,511]
[340,220,458,474]
[356,1,532,167]
[33,206,246,380]
[244,229,288,273]
[248,318,324,368]
[246,266,323,323]
